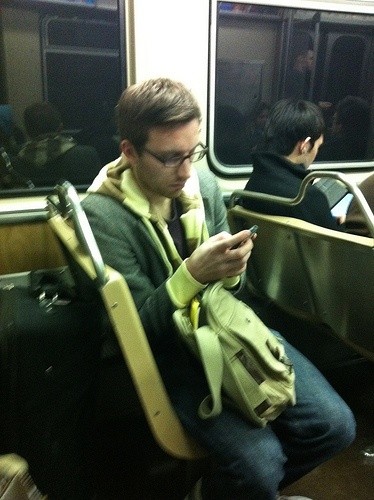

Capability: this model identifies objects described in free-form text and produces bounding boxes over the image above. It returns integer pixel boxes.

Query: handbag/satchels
[171,280,298,429]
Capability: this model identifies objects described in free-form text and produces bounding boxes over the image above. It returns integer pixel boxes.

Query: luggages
[0,268,143,500]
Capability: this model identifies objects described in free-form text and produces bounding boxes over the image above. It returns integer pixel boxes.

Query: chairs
[42,180,199,460]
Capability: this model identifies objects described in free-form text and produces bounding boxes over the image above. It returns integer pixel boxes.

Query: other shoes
[182,472,206,500]
[273,491,315,500]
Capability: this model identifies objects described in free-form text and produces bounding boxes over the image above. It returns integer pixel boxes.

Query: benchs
[230,169,374,358]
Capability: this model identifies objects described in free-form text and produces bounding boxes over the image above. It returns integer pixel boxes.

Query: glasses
[120,134,210,169]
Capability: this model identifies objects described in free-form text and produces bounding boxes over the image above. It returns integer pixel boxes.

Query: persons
[236,94,345,236]
[68,78,356,500]
[9,38,374,184]
